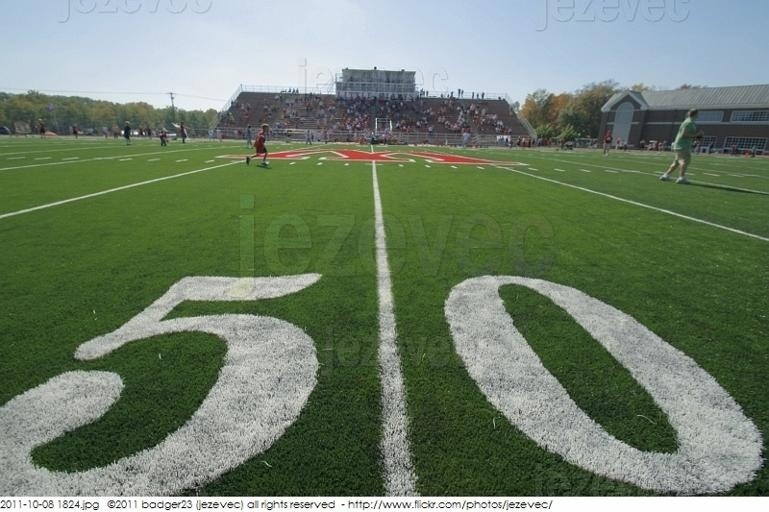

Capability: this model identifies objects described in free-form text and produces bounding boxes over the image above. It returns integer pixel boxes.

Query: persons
[657,108,703,187]
[537,129,769,159]
[34,117,193,148]
[244,123,272,168]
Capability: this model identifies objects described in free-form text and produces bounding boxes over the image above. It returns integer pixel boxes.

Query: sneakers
[261,162,269,166]
[246,156,251,165]
[660,173,670,181]
[675,176,690,184]
